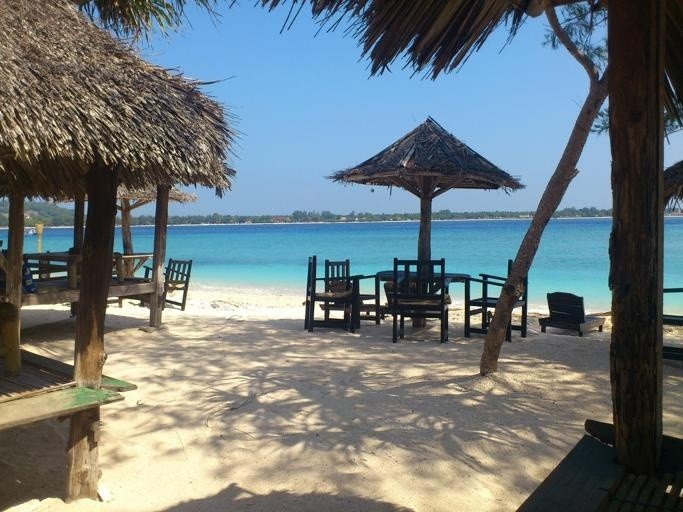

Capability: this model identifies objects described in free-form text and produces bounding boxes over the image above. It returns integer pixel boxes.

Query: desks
[375,270,470,328]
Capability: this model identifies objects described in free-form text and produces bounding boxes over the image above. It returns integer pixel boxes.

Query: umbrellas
[253,0,681,474]
[56,175,198,254]
[0,2,243,380]
[325,114,524,278]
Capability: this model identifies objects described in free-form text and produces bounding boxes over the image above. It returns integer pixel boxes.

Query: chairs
[138,258,192,311]
[303,255,380,333]
[392,257,527,344]
[538,292,605,337]
